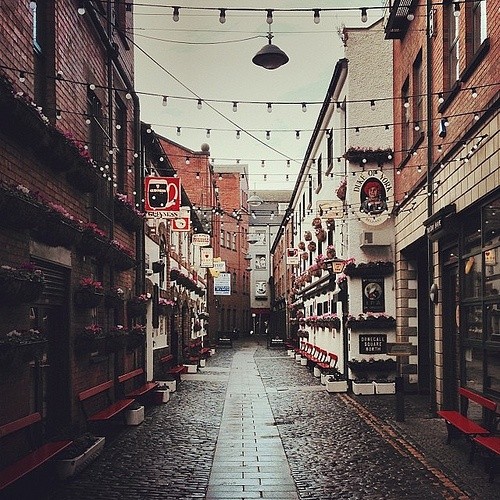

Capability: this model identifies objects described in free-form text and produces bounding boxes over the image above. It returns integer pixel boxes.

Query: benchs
[117,368,159,402]
[78,379,136,433]
[0,412,74,491]
[160,354,186,383]
[437,387,500,484]
[296,341,337,374]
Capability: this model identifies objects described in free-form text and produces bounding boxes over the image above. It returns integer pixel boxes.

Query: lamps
[430,283,438,303]
[251,35,289,69]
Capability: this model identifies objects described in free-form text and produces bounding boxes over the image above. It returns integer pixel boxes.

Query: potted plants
[178,359,197,374]
[153,383,170,403]
[288,348,301,360]
[200,357,206,367]
[155,377,176,392]
[321,371,335,385]
[54,435,106,475]
[126,403,144,426]
[314,365,330,377]
[373,377,395,394]
[300,356,307,365]
[326,375,348,392]
[352,377,374,395]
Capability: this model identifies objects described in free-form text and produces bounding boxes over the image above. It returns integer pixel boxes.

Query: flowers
[0,89,150,339]
[290,218,395,327]
